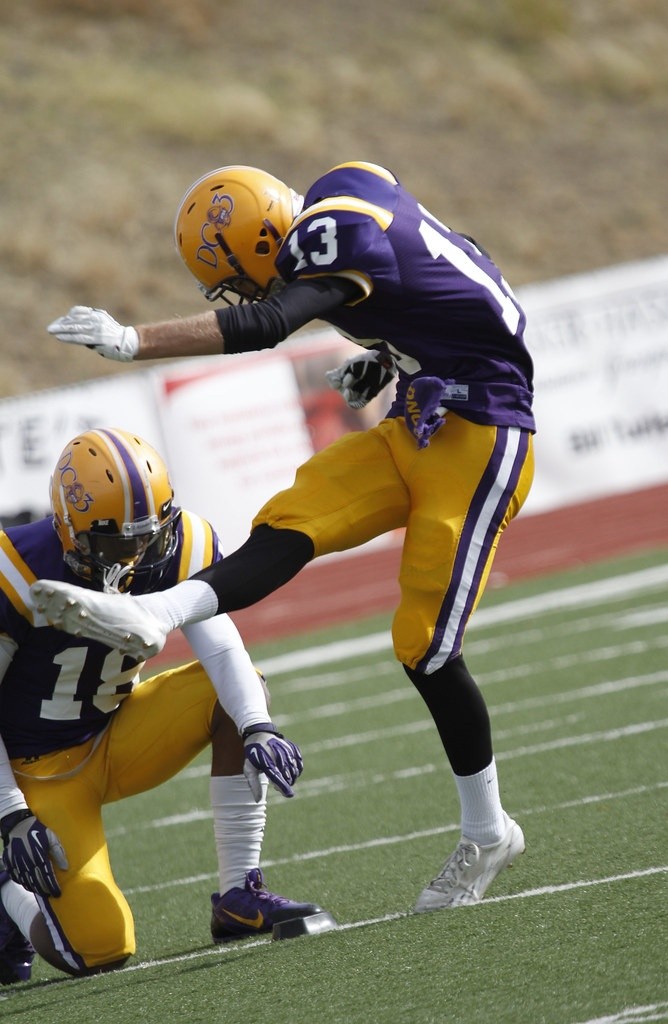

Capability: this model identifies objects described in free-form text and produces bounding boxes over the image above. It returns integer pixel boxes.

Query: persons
[1,430,326,984]
[30,162,536,915]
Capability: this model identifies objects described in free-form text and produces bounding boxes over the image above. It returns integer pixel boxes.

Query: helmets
[48,425,180,592]
[175,164,318,303]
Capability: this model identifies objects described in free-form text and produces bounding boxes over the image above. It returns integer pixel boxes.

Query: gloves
[241,722,309,803]
[323,349,398,410]
[46,305,142,363]
[0,808,78,899]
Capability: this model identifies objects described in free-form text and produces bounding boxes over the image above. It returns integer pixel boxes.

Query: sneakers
[30,579,166,661]
[0,900,37,983]
[414,818,527,913]
[209,867,324,943]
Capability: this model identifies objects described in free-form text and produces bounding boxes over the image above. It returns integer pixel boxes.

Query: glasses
[90,523,173,563]
